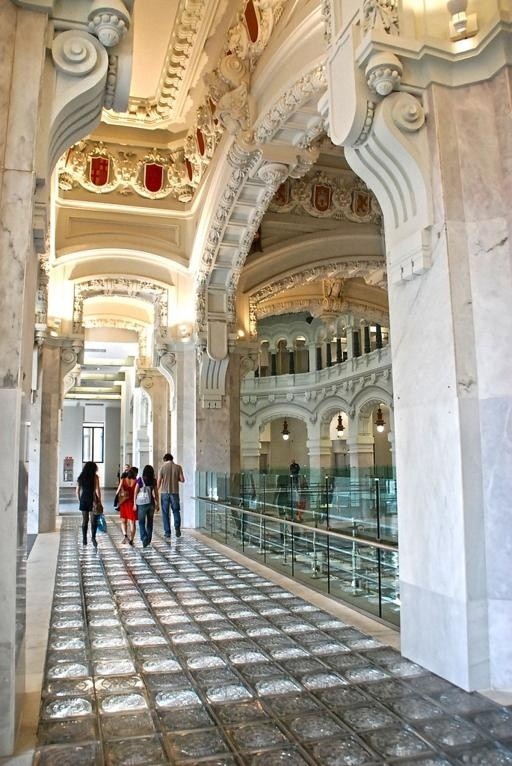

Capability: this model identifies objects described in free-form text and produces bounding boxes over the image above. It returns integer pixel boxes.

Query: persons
[116,467,139,544]
[76,461,101,547]
[157,452,185,537]
[289,459,300,484]
[132,465,160,547]
[115,464,131,511]
[229,474,336,540]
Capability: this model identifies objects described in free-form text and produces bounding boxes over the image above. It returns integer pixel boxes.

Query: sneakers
[164,532,170,537]
[143,536,148,547]
[175,526,181,537]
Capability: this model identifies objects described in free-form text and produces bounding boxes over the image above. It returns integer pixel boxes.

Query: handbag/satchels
[136,477,152,506]
[92,491,103,516]
[118,478,130,504]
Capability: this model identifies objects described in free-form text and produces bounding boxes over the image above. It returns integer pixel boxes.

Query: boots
[82,526,88,545]
[92,527,97,547]
